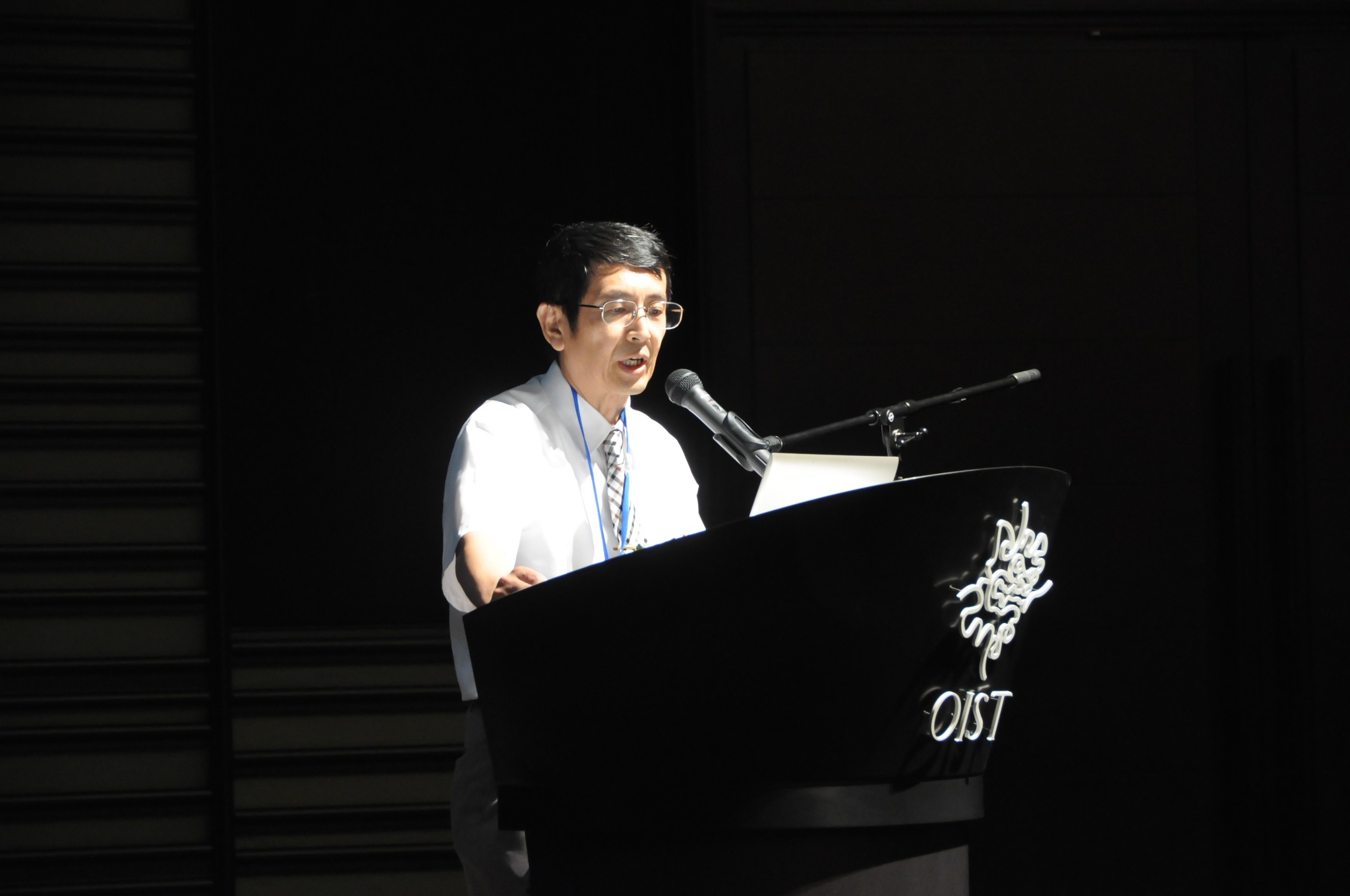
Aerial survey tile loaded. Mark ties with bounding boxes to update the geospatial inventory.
[602,431,647,555]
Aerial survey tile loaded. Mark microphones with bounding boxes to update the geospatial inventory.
[665,368,771,478]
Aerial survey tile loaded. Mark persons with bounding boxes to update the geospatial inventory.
[439,220,708,896]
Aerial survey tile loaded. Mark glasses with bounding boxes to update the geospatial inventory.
[554,300,684,330]
